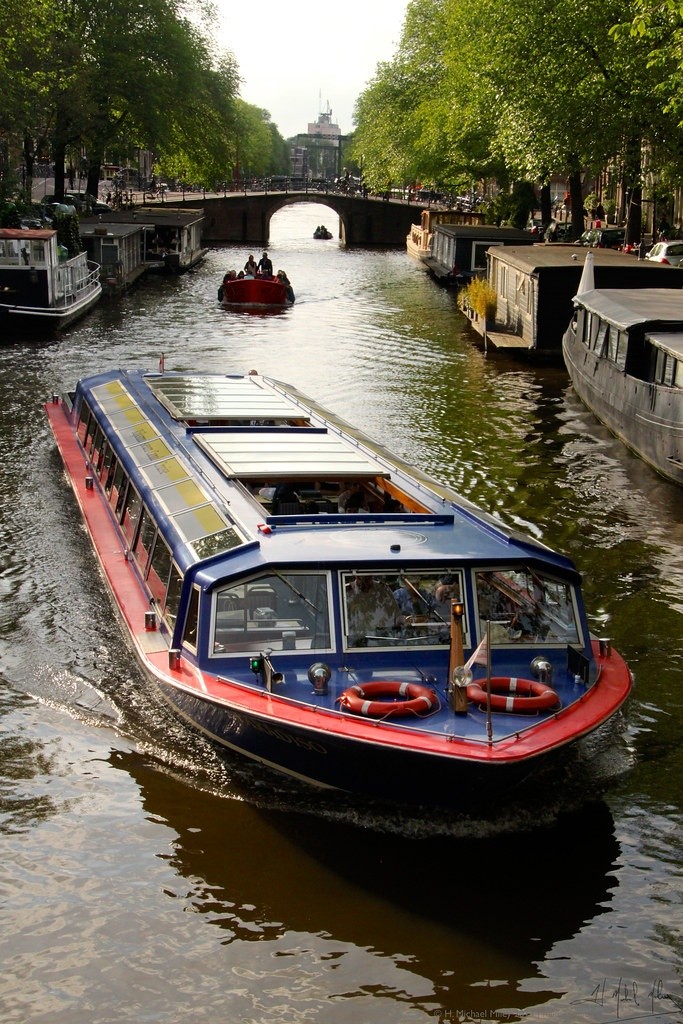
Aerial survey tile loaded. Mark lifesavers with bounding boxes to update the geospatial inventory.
[469,678,558,711]
[342,681,435,716]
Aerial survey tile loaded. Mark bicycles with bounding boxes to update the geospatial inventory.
[100,172,166,206]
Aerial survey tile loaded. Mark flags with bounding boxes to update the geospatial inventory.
[464,634,488,670]
[159,355,162,372]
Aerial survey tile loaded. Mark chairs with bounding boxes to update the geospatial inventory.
[274,498,303,514]
[215,585,278,623]
[308,498,331,513]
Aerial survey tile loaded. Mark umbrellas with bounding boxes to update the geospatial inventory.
[574,251,595,307]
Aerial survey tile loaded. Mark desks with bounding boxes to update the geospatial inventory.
[252,494,342,508]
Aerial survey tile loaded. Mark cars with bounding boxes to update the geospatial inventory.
[577,227,626,251]
[525,218,575,244]
[645,239,683,269]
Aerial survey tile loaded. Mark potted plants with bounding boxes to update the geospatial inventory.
[475,285,498,331]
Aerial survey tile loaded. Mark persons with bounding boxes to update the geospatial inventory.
[274,482,310,513]
[256,253,273,276]
[657,218,669,242]
[343,167,353,181]
[106,189,129,204]
[316,226,327,234]
[564,194,571,214]
[338,483,368,513]
[457,202,462,210]
[590,202,604,219]
[348,570,498,630]
[244,255,258,274]
[277,270,287,285]
[221,270,245,284]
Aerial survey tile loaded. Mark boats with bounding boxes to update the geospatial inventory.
[1,227,104,336]
[48,346,638,782]
[565,287,683,482]
[215,272,289,313]
